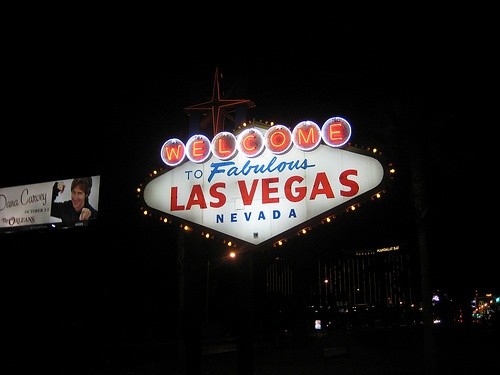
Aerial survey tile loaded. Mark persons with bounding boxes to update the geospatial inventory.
[51,176,98,222]
